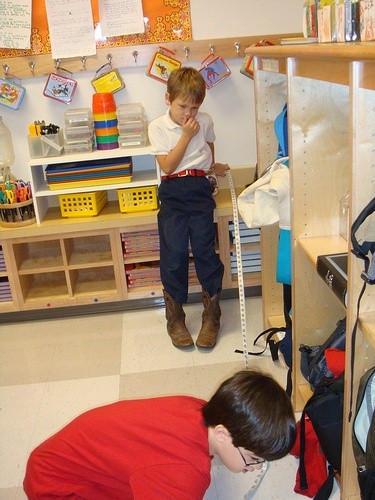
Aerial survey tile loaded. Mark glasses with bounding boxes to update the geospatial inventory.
[230,431,266,469]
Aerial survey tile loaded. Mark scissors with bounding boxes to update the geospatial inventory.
[0,180,35,223]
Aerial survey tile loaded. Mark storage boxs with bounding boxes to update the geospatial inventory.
[59,190,109,218]
[116,185,158,213]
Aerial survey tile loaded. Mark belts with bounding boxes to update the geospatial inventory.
[161,169,207,180]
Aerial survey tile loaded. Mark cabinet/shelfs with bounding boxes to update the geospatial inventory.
[0,166,262,314]
[28,143,161,223]
[243,39,375,500]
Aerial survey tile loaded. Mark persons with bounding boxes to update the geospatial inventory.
[23,367,297,500]
[148,67,231,348]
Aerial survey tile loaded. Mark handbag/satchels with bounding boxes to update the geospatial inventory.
[234,327,292,371]
[298,316,345,388]
[352,366,375,500]
[299,372,344,500]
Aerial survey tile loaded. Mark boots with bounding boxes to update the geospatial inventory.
[162,289,194,347]
[196,289,222,348]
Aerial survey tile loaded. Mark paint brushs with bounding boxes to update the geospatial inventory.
[40,124,59,134]
[28,120,45,136]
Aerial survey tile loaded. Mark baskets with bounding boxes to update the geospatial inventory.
[115,185,158,212]
[58,189,108,217]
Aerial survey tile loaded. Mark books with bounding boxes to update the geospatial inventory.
[230,249,261,273]
[316,252,349,309]
[281,0,375,44]
[228,220,260,245]
[0,282,13,302]
[121,228,217,288]
[0,251,6,272]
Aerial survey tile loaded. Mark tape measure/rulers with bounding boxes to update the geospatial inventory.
[227,168,269,497]
